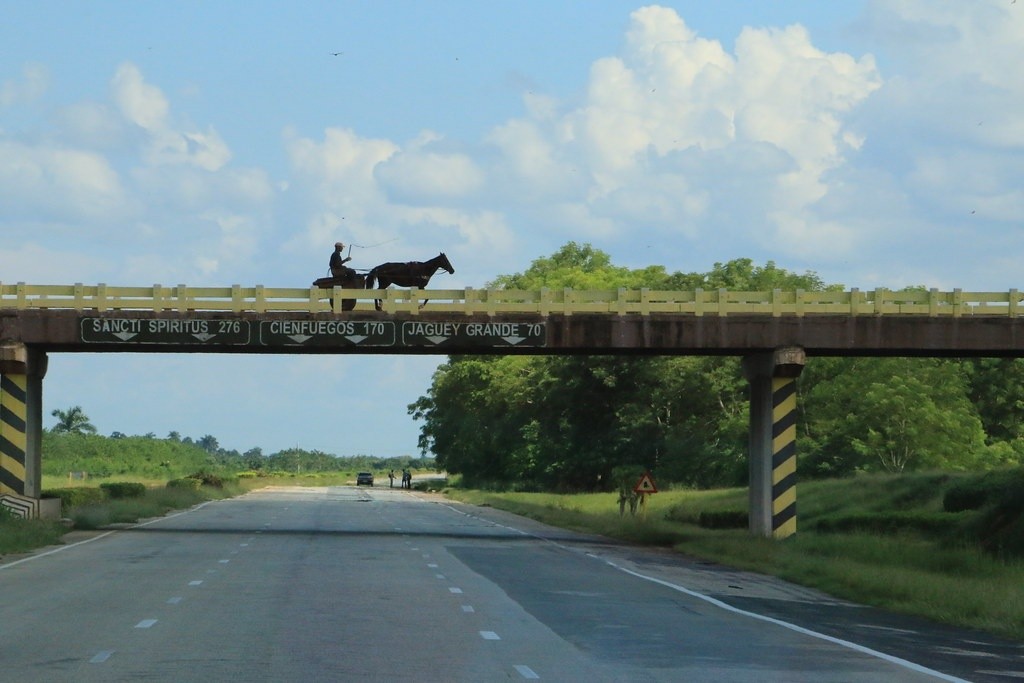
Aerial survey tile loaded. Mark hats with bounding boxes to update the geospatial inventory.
[335,242,346,248]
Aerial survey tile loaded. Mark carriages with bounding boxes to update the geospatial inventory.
[313,252,455,311]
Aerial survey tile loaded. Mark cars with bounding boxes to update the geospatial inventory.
[357,473,374,487]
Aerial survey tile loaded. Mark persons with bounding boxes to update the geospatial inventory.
[401,469,411,489]
[329,242,355,281]
[389,469,396,488]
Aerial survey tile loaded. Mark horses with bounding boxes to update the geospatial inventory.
[365,252,455,311]
